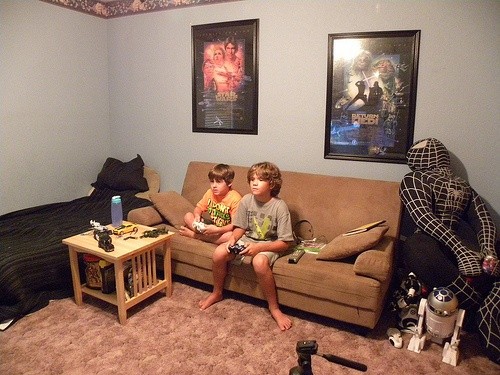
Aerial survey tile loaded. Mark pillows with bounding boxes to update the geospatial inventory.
[91,159,149,192]
[149,191,195,229]
[317,226,390,259]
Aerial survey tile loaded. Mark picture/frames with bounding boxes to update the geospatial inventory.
[323,29,422,165]
[191,19,260,135]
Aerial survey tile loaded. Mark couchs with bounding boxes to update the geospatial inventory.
[127,161,405,330]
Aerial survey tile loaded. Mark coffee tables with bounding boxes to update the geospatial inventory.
[63,221,176,324]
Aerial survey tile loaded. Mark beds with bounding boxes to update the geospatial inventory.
[0,167,161,332]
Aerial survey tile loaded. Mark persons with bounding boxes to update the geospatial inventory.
[202,35,243,93]
[199,162,296,330]
[400,136,500,365]
[179,164,243,245]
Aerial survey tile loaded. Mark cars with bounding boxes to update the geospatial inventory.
[113,224,134,235]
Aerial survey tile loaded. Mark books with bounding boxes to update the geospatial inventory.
[342,220,386,236]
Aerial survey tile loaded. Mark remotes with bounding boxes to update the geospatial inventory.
[287,249,305,263]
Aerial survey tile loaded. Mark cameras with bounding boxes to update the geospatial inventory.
[98,232,115,251]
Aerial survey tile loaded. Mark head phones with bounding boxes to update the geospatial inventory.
[292,219,316,246]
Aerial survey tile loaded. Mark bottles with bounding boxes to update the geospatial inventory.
[110,195,122,227]
